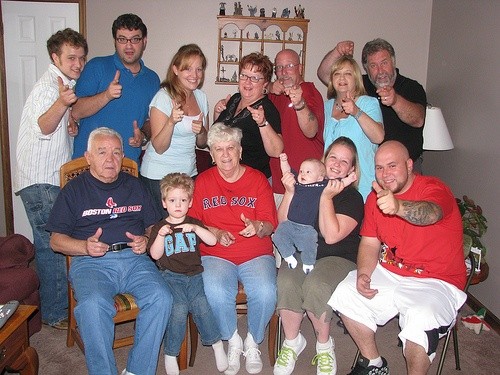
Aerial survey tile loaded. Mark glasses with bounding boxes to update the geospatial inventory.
[239,73,264,83]
[274,64,298,72]
[116,36,142,44]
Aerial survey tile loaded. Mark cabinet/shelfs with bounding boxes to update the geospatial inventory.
[215,15,310,85]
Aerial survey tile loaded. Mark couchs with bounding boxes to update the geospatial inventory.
[0,234,40,337]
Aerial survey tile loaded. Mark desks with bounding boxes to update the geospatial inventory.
[0,305,38,375]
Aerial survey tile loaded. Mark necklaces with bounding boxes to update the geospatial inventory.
[131,62,141,74]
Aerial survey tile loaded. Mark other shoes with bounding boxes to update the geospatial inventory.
[43,319,69,329]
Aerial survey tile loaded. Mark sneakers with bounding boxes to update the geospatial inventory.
[273,331,307,375]
[312,336,337,375]
[224,336,244,375]
[347,354,391,375]
[243,341,263,374]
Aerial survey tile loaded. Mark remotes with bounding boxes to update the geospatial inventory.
[0,300,19,327]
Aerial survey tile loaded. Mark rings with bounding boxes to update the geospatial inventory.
[226,244,228,245]
[218,107,220,109]
[293,95,295,98]
[247,232,249,235]
[385,97,387,101]
[139,248,141,251]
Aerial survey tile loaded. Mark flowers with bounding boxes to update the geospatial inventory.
[456,195,488,264]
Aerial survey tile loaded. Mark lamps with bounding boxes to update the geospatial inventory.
[422,106,455,150]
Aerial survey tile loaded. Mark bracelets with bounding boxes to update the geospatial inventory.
[355,110,362,119]
[294,100,305,110]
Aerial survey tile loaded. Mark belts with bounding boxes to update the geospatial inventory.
[106,243,132,253]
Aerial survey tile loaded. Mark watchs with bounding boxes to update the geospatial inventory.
[258,121,269,127]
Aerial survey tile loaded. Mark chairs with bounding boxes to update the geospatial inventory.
[351,251,475,375]
[189,283,279,367]
[61,158,188,370]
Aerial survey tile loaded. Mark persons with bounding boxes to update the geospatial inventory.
[272,152,358,274]
[45,127,173,375]
[317,37,427,170]
[70,13,161,170]
[273,136,365,375]
[328,141,468,375]
[140,44,209,198]
[267,49,324,209]
[15,28,89,330]
[321,55,385,204]
[186,122,279,375]
[212,51,284,190]
[147,173,229,375]
